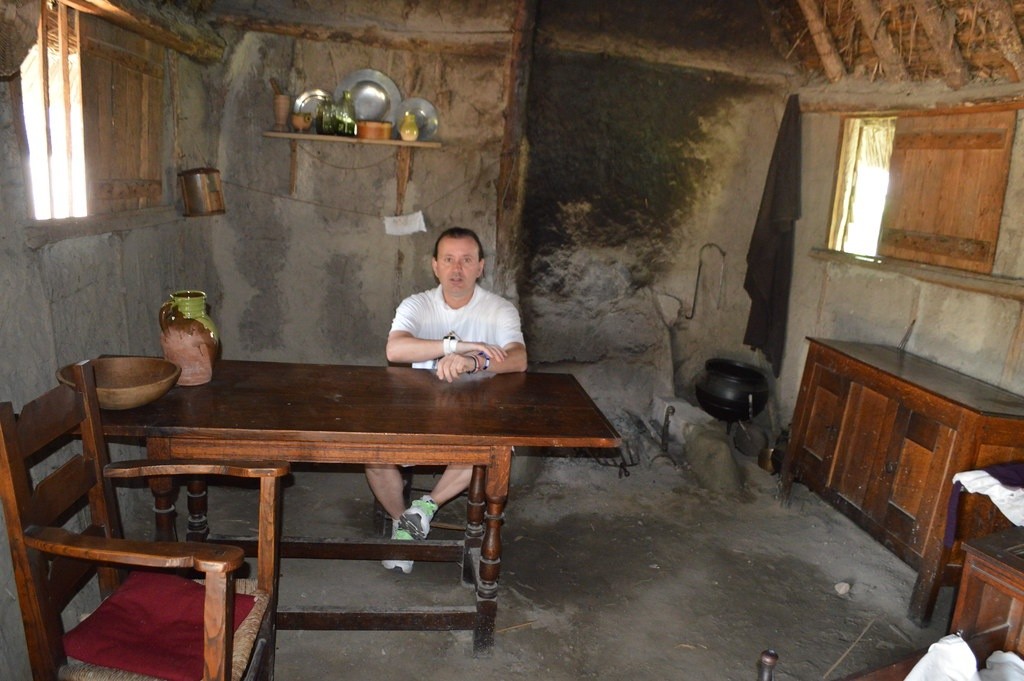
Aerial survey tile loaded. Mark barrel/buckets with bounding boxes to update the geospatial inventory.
[177,167,225,217]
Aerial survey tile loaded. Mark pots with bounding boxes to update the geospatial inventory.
[695,358,770,436]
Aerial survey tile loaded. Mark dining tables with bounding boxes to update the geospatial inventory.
[74,352,624,652]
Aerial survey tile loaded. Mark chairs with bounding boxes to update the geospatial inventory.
[0,362,279,681]
[375,331,483,541]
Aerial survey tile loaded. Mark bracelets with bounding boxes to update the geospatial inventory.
[443,333,490,375]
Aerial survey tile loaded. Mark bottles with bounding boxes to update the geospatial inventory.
[335,88,358,136]
[769,427,790,476]
[159,289,220,386]
[290,112,312,133]
[398,112,419,142]
[272,93,290,132]
[315,95,337,135]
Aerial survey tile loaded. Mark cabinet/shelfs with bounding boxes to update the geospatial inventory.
[770,335,1023,629]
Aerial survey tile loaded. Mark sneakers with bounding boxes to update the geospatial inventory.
[398,494,437,541]
[383,521,416,573]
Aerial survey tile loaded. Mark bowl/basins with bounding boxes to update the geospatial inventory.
[56,357,182,410]
[356,120,392,138]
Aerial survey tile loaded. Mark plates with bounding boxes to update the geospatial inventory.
[293,89,337,133]
[331,68,402,139]
[396,97,439,142]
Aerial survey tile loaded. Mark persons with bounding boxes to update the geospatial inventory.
[366,227,530,576]
